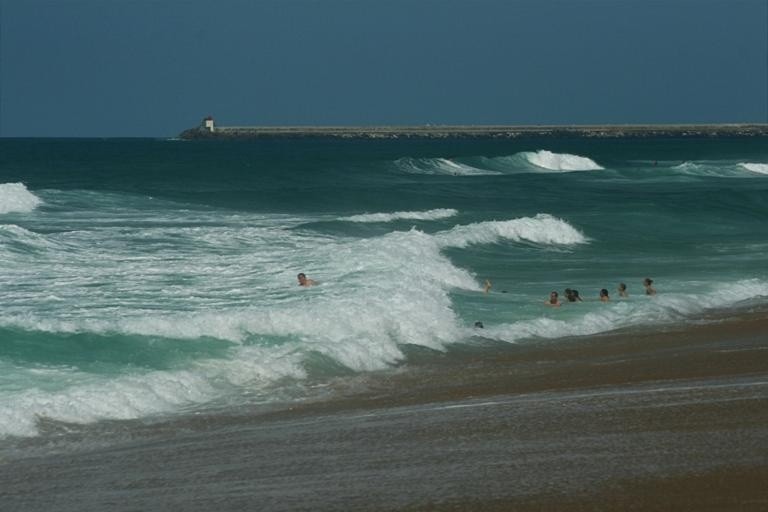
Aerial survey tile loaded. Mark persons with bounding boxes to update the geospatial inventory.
[546,277,584,308]
[473,321,484,328]
[618,283,629,298]
[295,273,317,288]
[600,288,610,303]
[485,279,492,293]
[642,278,655,295]
